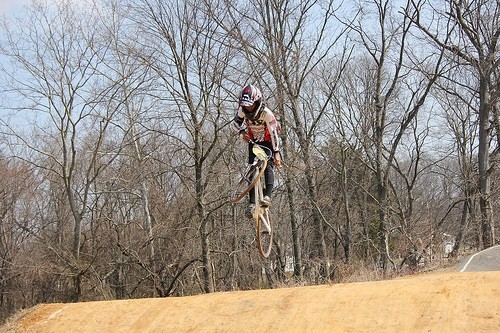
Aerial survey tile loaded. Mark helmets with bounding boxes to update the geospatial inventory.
[239,84,262,118]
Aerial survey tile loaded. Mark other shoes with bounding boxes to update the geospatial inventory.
[244,203,255,214]
[261,195,270,205]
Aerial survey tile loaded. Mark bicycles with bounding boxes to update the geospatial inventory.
[229,139,289,260]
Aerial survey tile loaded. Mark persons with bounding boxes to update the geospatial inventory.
[233,85,282,218]
